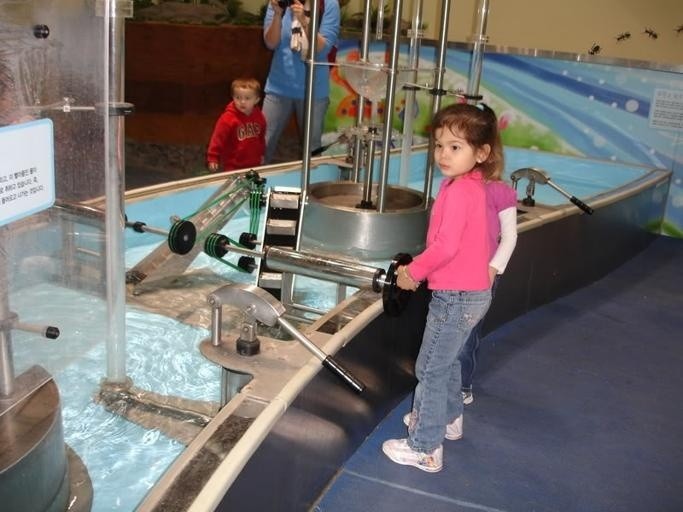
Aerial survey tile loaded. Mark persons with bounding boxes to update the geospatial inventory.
[206,76,267,171]
[459,131,517,404]
[262,0,340,165]
[382,103,497,472]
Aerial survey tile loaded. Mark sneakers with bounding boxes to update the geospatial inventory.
[382,439,443,472]
[461,391,473,404]
[403,412,463,440]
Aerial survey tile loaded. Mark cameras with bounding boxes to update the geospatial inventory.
[278,0,305,11]
